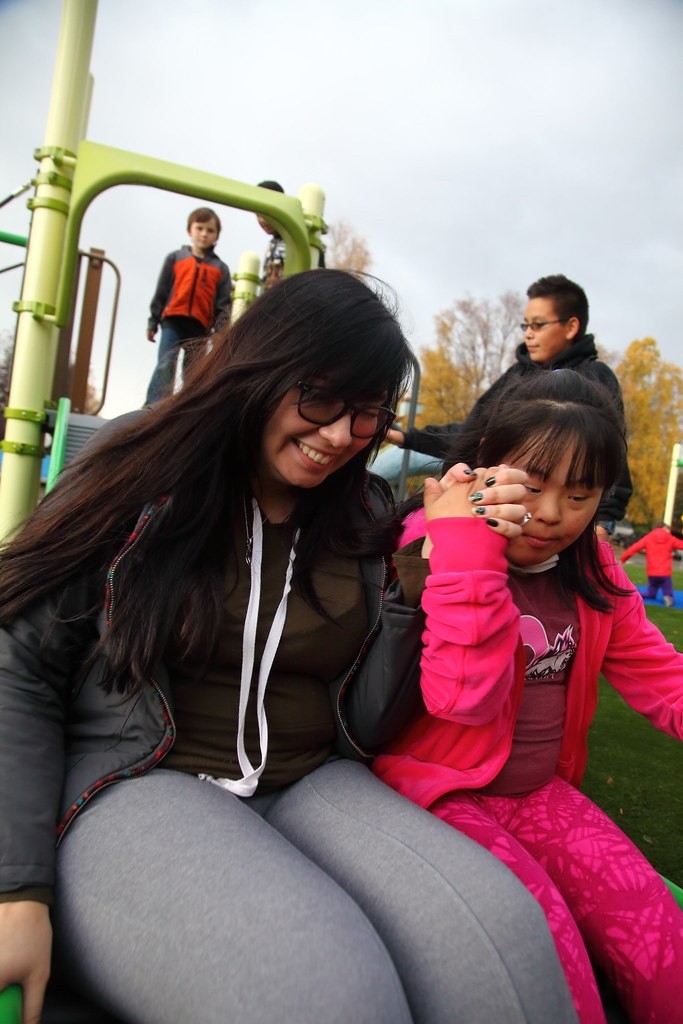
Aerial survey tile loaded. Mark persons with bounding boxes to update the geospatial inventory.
[144,208,232,409]
[384,275,633,541]
[363,369,682,1024]
[254,181,286,287]
[0,270,577,1024]
[620,521,683,606]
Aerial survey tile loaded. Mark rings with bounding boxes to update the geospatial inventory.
[520,512,531,525]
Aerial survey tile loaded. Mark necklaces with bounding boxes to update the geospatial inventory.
[243,495,272,566]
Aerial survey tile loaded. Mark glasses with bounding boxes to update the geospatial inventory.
[520,318,571,332]
[293,379,395,441]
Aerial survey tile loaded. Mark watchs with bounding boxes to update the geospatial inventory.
[597,520,614,535]
